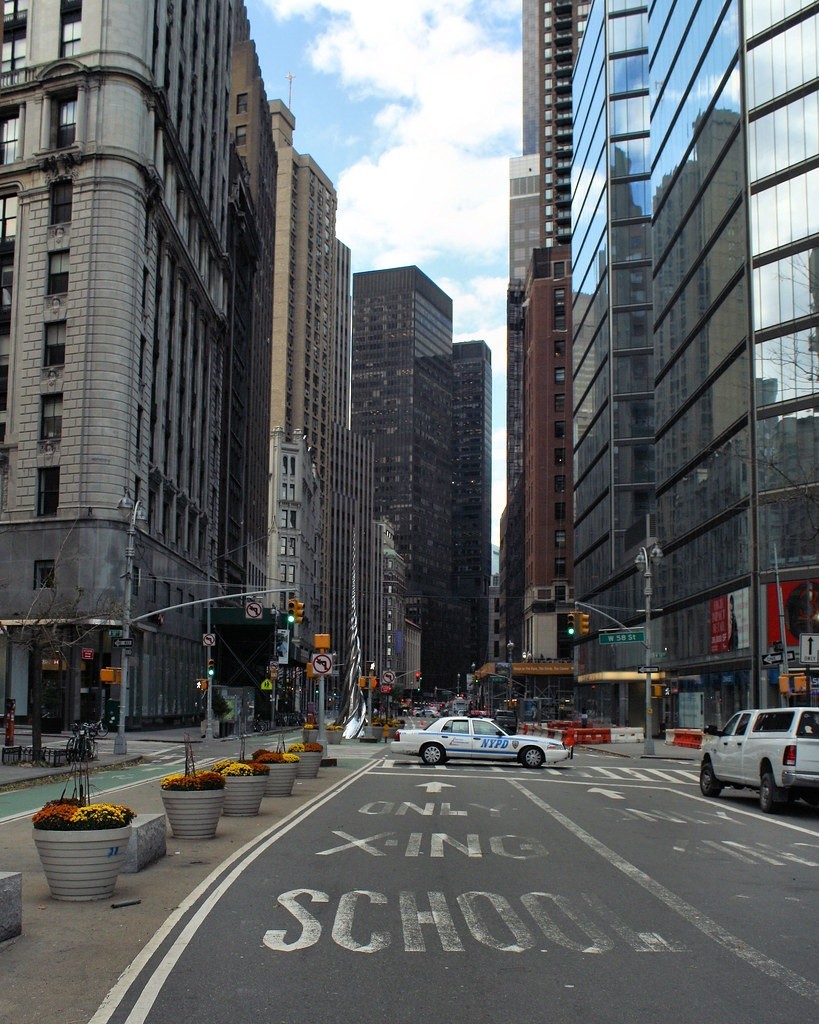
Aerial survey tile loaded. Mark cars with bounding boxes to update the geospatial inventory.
[413,702,467,716]
[390,716,573,769]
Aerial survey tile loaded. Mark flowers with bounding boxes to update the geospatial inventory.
[380,718,405,728]
[211,759,271,776]
[289,741,324,754]
[304,723,319,730]
[365,717,383,728]
[250,749,300,763]
[160,770,227,792]
[33,798,138,833]
[326,725,345,731]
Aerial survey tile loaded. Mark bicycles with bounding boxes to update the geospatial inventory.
[66,712,108,762]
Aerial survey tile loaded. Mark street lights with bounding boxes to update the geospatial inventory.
[471,663,475,701]
[635,540,664,755]
[456,674,460,693]
[506,640,515,708]
[269,602,279,729]
[114,486,147,754]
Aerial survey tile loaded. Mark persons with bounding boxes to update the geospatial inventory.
[531,703,537,721]
[379,706,385,718]
[728,595,739,652]
[582,704,588,728]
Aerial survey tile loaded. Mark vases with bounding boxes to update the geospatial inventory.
[261,763,298,797]
[326,730,344,745]
[289,752,322,778]
[160,787,227,838]
[387,728,399,738]
[33,826,132,902]
[221,775,268,817]
[302,730,319,742]
[398,724,405,729]
[364,727,384,741]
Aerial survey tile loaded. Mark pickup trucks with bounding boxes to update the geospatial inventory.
[494,710,518,732]
[700,707,819,814]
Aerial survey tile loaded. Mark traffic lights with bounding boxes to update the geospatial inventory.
[457,693,465,697]
[778,676,789,694]
[567,614,575,636]
[416,673,420,681]
[420,672,423,681]
[208,659,215,678]
[287,600,295,624]
[653,685,662,697]
[578,613,590,634]
[358,677,365,687]
[365,661,375,670]
[194,680,207,690]
[296,600,305,623]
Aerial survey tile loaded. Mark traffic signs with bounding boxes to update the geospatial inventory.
[762,650,795,664]
[112,637,133,647]
[637,666,660,673]
[663,687,673,697]
